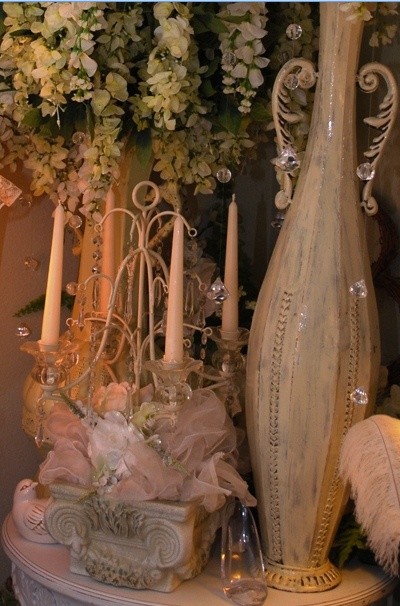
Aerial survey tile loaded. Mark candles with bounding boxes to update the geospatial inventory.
[99,182,117,313]
[220,196,242,334]
[161,210,189,368]
[39,200,65,345]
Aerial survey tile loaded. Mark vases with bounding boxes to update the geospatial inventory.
[244,2,398,594]
[22,141,161,494]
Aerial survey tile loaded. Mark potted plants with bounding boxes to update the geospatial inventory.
[0,1,400,349]
